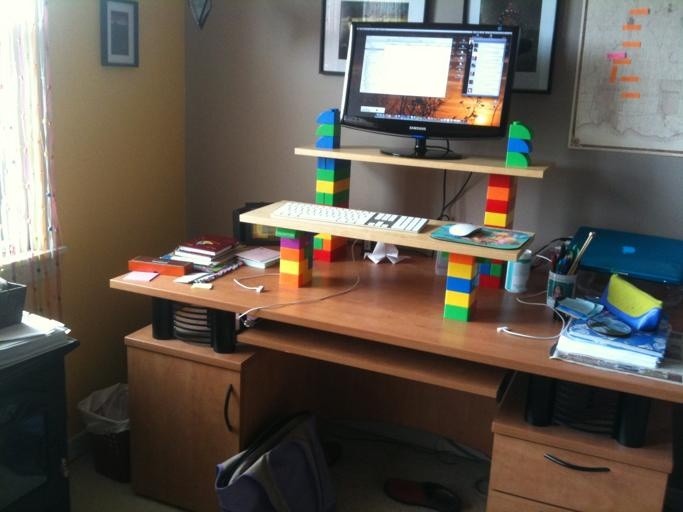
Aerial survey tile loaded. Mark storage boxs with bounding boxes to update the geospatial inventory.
[571,225,682,306]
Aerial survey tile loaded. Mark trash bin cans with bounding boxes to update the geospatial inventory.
[78,384,133,482]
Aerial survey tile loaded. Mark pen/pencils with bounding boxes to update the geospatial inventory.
[192,261,242,282]
[548,242,580,275]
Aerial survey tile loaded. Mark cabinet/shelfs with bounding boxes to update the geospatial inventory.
[483,434,671,511]
[126,345,308,511]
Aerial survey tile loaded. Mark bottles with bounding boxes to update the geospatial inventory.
[504,248,533,293]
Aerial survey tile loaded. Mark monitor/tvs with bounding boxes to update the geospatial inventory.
[339,21,522,160]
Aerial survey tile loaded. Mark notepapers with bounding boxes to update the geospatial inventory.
[234,246,279,269]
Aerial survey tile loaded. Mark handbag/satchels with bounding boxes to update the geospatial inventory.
[215,410,338,511]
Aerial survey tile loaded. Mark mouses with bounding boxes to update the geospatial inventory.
[449,222,481,237]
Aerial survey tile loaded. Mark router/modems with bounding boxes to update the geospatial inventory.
[360,241,376,257]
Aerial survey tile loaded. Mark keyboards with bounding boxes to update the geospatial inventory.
[272,200,430,234]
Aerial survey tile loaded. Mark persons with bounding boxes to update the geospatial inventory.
[551,284,564,300]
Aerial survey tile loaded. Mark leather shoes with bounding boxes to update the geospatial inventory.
[382,478,461,511]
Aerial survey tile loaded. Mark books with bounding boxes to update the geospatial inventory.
[557,316,663,369]
[159,234,282,273]
[566,311,672,357]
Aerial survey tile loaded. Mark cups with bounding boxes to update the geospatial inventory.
[546,268,580,308]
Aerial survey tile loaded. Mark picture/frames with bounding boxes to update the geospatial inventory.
[318,1,559,96]
[98,1,140,67]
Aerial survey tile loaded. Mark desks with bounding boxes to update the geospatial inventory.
[109,238,683,511]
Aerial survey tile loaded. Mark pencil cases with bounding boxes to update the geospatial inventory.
[128,256,193,276]
